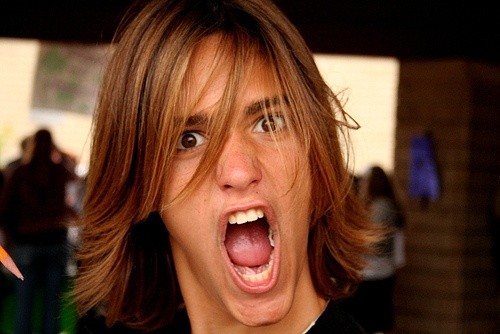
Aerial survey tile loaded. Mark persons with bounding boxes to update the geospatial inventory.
[346,166,406,334]
[0,129,78,333]
[71,1,399,334]
